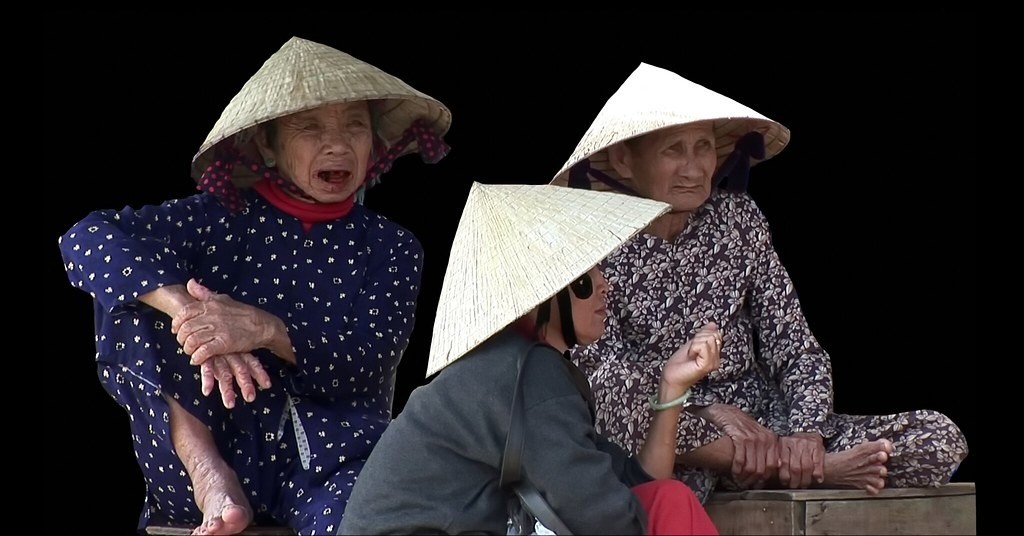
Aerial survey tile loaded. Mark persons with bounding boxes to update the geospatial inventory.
[59,37,452,536]
[337,181,722,535]
[548,62,968,507]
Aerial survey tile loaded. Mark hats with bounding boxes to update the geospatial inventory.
[552,60,790,193]
[425,181,673,383]
[192,37,452,189]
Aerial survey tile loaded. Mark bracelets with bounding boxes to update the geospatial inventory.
[649,390,690,410]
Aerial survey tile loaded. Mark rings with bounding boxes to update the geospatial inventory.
[716,339,721,345]
